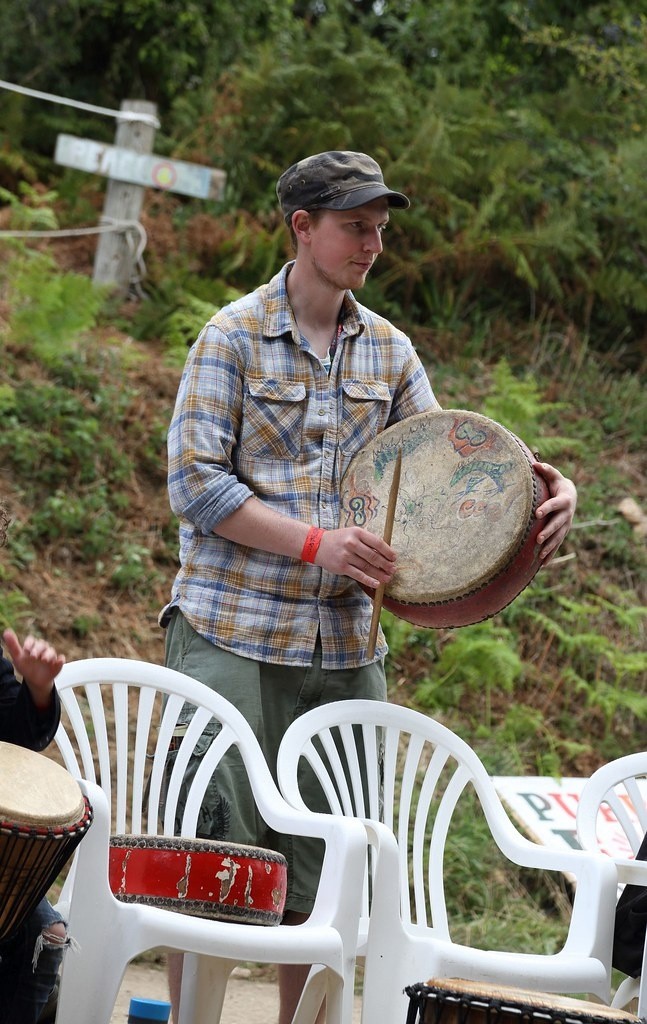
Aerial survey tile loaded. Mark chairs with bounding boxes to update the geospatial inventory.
[49,657,647,1024]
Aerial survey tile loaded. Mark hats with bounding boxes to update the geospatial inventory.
[276,150,410,220]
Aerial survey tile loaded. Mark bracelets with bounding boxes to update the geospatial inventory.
[297,524,325,564]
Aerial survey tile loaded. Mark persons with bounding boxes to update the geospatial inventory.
[158,149,576,1024]
[0,627,71,1024]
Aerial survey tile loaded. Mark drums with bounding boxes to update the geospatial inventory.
[340,406,557,630]
[405,977,645,1024]
[0,739,93,958]
[109,834,287,928]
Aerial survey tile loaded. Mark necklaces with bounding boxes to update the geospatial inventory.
[286,286,341,379]
